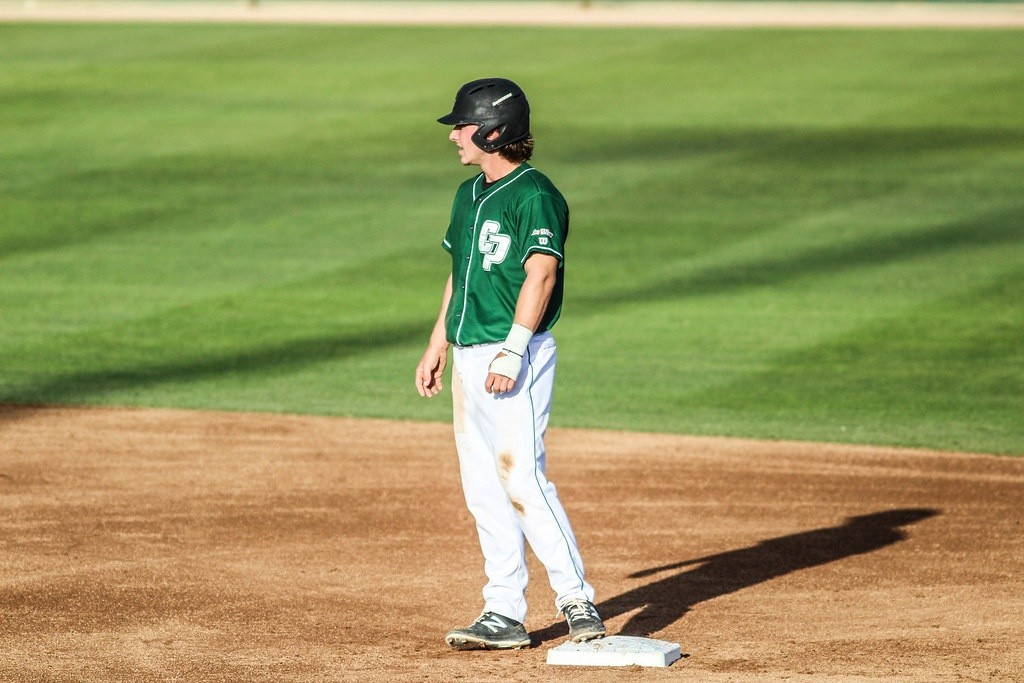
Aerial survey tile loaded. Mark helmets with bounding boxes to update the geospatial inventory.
[436,78,530,153]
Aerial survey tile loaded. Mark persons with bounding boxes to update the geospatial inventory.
[414,78,608,651]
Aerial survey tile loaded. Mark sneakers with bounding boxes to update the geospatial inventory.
[556,598,606,643]
[445,612,531,650]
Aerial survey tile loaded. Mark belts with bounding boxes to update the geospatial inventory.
[455,343,472,347]
